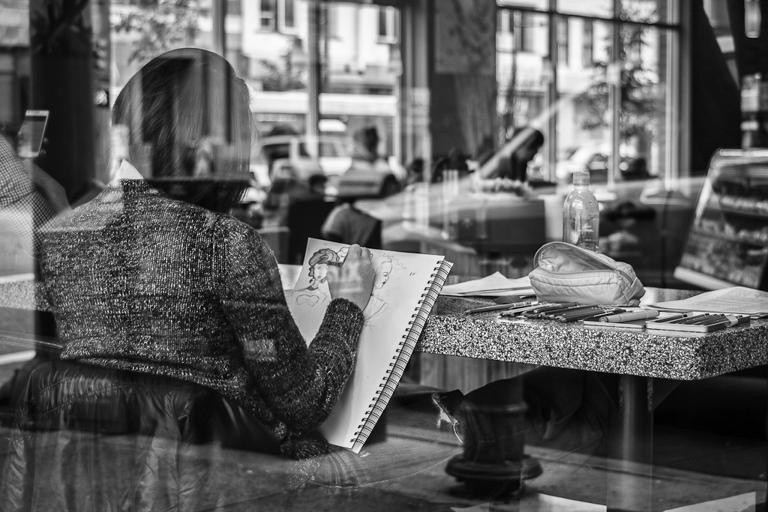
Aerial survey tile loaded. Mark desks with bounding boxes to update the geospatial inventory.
[414,276,768,512]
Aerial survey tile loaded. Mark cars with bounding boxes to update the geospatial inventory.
[357,67,684,277]
[250,133,407,204]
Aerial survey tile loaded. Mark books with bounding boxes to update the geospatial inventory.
[287,236,454,456]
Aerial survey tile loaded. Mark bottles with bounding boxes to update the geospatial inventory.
[563,172,601,253]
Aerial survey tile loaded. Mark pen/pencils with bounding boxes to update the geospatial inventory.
[327,261,342,267]
[457,286,531,294]
[463,299,743,326]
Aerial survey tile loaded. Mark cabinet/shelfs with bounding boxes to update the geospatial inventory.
[673,147,768,290]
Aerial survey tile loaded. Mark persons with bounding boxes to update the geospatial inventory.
[0,135,72,259]
[31,43,378,458]
[317,124,408,202]
[472,124,545,180]
[362,255,393,321]
[306,248,341,291]
